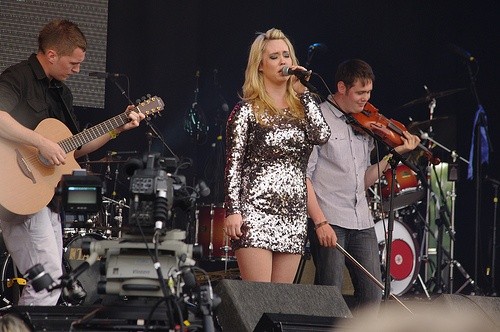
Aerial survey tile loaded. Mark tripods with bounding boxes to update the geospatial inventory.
[419,185,485,299]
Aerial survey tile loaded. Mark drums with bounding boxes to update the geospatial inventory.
[371,217,421,297]
[0,207,111,313]
[375,161,427,212]
[197,202,239,264]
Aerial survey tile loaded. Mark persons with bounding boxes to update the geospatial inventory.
[225,26,332,284]
[308,60,421,307]
[0,18,146,306]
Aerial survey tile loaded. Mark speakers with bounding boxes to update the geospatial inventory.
[211,278,355,332]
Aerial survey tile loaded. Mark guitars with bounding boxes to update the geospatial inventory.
[0,93,165,217]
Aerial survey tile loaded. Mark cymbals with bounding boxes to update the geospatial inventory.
[79,155,129,164]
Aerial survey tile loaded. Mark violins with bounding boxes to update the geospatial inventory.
[348,101,440,165]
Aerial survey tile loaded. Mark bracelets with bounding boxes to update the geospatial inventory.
[383,156,390,161]
[109,130,117,138]
[315,221,328,230]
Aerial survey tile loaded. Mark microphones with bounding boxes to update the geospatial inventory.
[90,72,122,78]
[282,66,317,76]
[198,181,210,197]
[462,49,477,64]
